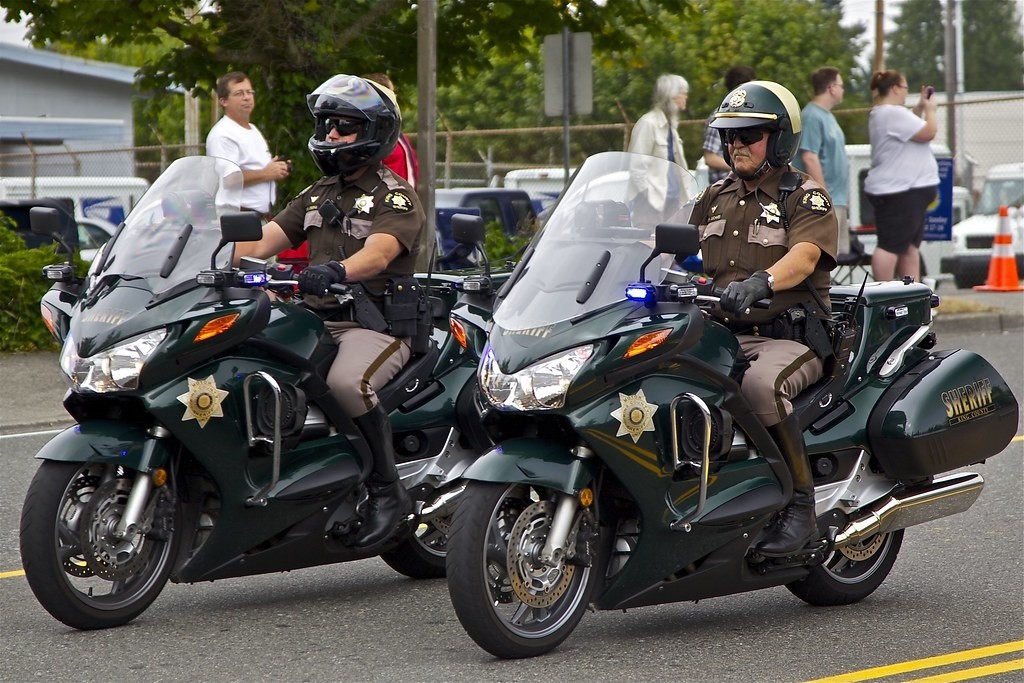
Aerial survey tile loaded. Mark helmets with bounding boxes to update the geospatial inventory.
[709,79,802,169]
[306,74,401,176]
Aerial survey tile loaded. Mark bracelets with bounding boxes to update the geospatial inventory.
[765,270,775,290]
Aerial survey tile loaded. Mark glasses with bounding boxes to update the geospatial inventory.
[232,88,255,98]
[726,129,770,145]
[834,82,843,88]
[323,118,363,136]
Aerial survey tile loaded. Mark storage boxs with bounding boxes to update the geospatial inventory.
[824,278,940,347]
[867,350,1018,480]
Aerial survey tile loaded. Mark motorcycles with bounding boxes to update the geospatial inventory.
[446,153,1018,658]
[17,153,506,631]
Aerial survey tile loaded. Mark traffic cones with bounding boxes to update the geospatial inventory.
[973,206,1024,291]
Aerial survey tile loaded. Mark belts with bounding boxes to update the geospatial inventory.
[317,305,356,321]
[725,323,794,339]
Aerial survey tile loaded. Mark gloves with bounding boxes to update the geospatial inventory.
[298,260,346,299]
[720,269,776,316]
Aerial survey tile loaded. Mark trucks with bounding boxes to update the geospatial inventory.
[0,176,149,260]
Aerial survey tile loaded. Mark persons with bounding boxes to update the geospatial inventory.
[613,81,838,557]
[791,66,850,255]
[863,71,941,317]
[231,74,426,552]
[206,71,292,265]
[624,74,689,236]
[363,73,419,192]
[703,65,756,185]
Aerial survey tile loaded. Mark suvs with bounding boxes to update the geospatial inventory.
[845,142,1024,289]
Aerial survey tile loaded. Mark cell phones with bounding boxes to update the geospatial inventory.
[927,86,934,99]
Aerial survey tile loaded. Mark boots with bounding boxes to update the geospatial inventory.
[352,401,415,554]
[755,410,821,557]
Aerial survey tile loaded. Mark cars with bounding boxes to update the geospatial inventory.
[434,167,577,267]
